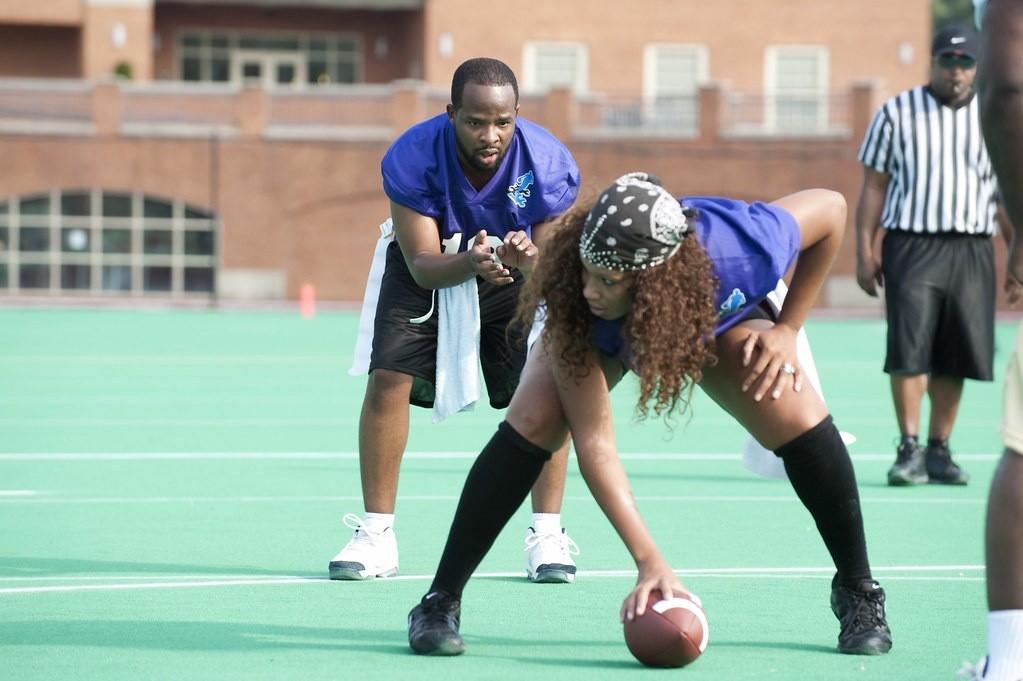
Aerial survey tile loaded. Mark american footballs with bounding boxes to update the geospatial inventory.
[624,588,710,669]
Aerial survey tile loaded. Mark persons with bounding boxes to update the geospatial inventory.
[395,167,897,656]
[327,58,584,588]
[961,1,1023,681]
[855,25,1023,488]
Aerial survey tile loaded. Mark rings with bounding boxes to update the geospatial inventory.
[778,363,795,375]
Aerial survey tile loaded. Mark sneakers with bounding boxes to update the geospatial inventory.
[328,514,399,579]
[831,572,893,655]
[409,589,467,657]
[890,439,968,485]
[524,525,581,583]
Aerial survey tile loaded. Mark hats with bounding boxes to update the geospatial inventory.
[579,170,695,272]
[931,23,979,60]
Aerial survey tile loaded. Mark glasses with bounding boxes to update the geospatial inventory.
[933,52,978,70]
